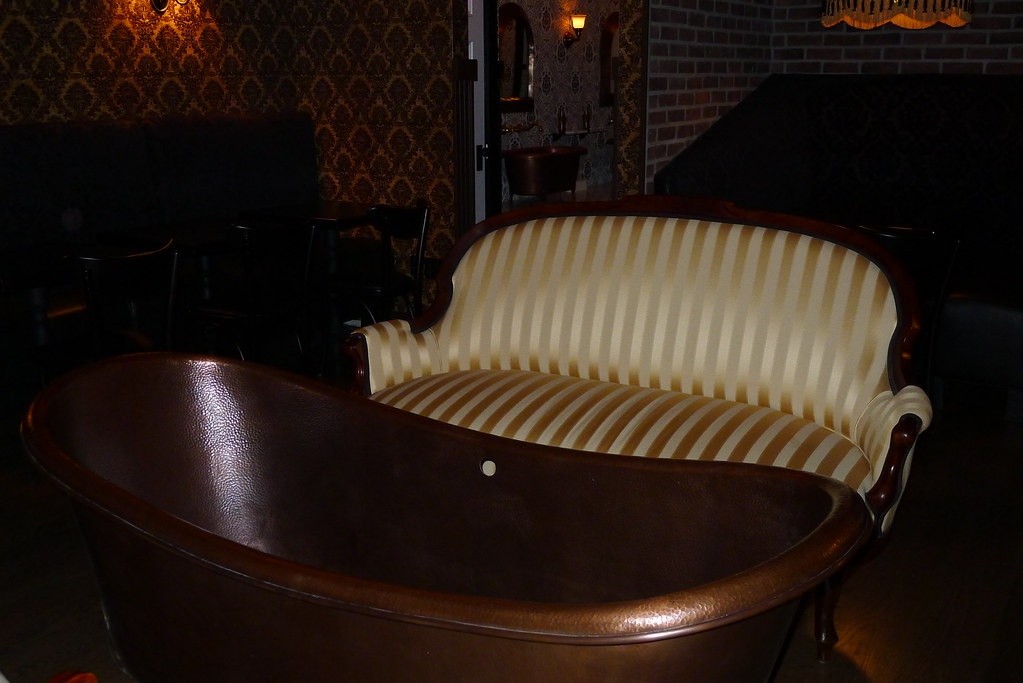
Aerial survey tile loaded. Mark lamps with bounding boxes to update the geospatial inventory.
[563,14,587,49]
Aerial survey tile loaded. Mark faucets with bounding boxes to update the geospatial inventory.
[519,112,544,133]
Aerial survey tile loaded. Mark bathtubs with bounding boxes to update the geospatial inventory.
[13,351,876,683]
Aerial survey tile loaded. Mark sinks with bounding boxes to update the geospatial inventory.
[503,145,588,197]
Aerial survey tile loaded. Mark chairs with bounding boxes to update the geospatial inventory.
[33,203,432,394]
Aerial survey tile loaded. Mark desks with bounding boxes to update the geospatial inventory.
[19,350,872,683]
[0,204,341,426]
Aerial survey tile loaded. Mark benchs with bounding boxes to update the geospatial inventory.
[342,197,933,669]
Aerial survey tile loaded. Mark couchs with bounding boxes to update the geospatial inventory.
[923,237,1023,424]
